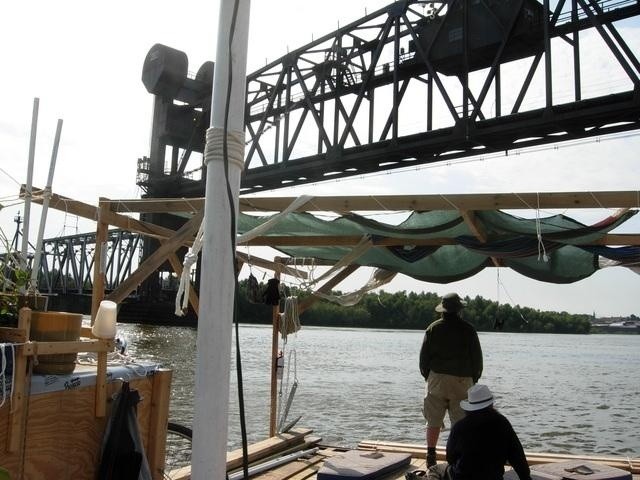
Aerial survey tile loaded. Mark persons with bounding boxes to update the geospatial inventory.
[406,383,532,480]
[418,293,484,470]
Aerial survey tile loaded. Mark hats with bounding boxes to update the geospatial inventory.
[460,385,502,412]
[434,292,468,313]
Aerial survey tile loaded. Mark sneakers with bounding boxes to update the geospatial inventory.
[426,452,436,468]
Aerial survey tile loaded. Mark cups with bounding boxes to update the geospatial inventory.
[91,300,118,340]
